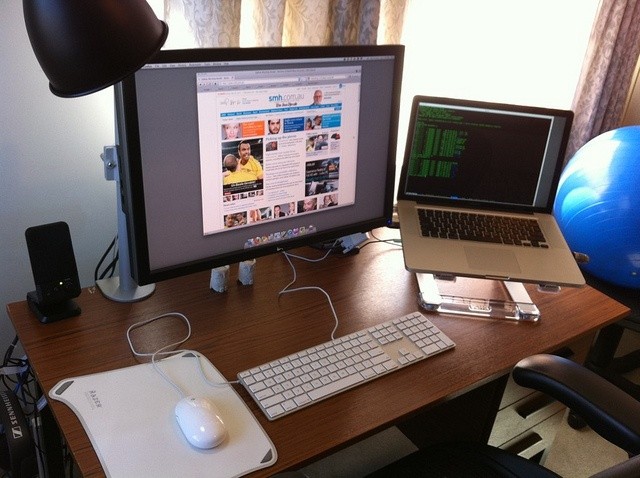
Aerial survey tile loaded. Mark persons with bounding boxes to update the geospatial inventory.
[328,193,337,206]
[310,90,324,105]
[237,213,246,226]
[223,154,257,186]
[306,136,316,152]
[237,142,262,179]
[273,205,285,219]
[223,123,240,140]
[305,119,312,129]
[314,115,322,127]
[225,215,236,228]
[315,135,330,151]
[303,198,316,211]
[319,196,331,208]
[268,119,280,135]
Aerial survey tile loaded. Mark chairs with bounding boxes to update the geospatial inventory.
[355,352,639,478]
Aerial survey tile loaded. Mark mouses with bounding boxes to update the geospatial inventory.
[174,394,229,450]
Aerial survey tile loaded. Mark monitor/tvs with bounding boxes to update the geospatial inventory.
[95,44,405,303]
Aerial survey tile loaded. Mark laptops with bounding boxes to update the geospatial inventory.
[396,95,586,288]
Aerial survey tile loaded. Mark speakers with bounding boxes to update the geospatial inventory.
[24,220,82,324]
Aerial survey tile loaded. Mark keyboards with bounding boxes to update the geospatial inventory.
[237,310,457,421]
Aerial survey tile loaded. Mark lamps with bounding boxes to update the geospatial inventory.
[22,0,170,99]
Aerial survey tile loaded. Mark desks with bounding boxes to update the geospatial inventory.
[6,218,631,478]
